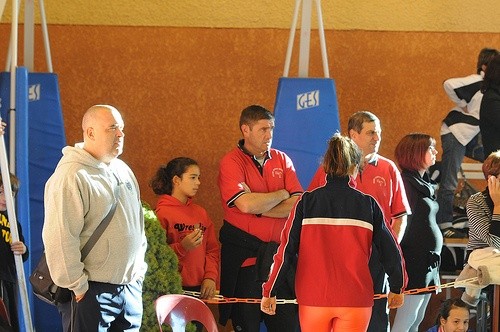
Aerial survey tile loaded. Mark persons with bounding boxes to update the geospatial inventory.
[479,54,500,159]
[425,299,470,332]
[391,133,443,332]
[218,105,304,332]
[463,149,500,332]
[260,132,409,332]
[152,157,220,332]
[320,111,413,332]
[0,118,29,332]
[436,48,500,239]
[42,104,148,332]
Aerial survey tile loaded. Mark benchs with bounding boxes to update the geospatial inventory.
[441,163,485,299]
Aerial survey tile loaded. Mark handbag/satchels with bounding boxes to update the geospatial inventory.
[30,249,78,307]
[454,165,478,211]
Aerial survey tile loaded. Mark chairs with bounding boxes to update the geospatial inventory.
[154,294,219,332]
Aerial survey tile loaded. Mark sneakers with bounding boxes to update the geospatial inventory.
[441,227,468,239]
